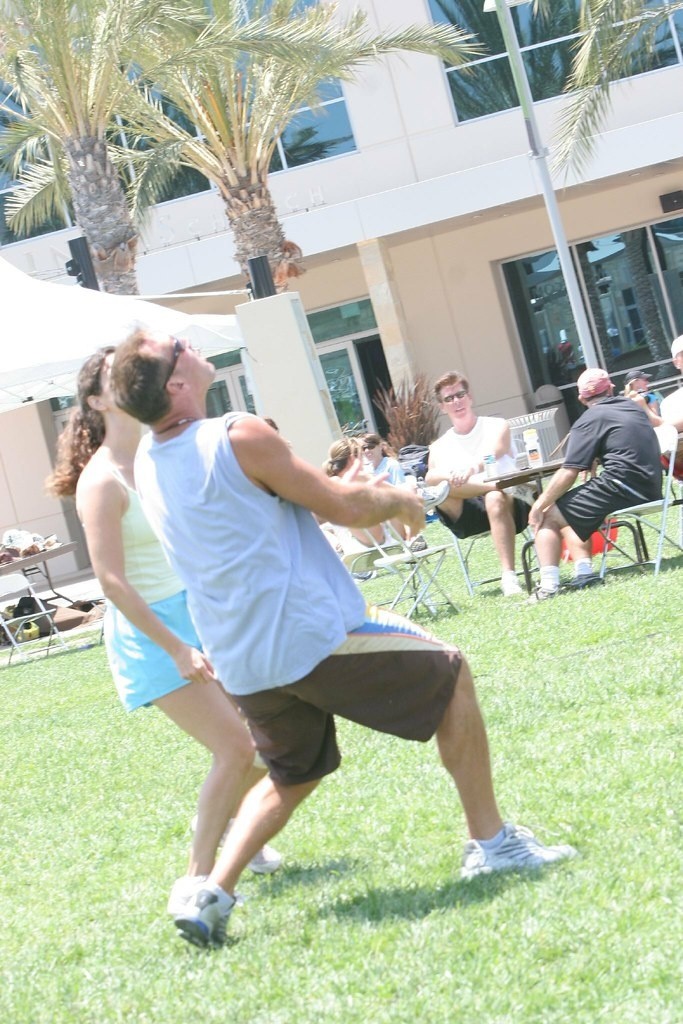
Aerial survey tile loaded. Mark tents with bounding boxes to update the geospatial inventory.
[0,256,263,422]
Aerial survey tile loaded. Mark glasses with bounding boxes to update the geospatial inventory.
[442,390,468,403]
[164,339,185,390]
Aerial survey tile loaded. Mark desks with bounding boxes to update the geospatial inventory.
[473,459,643,595]
[0,541,93,651]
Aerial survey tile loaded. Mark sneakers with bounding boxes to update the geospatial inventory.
[190,810,282,874]
[174,885,237,950]
[461,825,578,878]
[168,877,209,917]
[415,480,451,513]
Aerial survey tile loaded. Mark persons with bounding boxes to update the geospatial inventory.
[319,433,428,564]
[106,327,577,951]
[425,371,540,596]
[525,367,663,604]
[44,345,281,919]
[660,335,683,471]
[624,370,662,425]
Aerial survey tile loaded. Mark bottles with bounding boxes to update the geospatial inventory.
[482,440,498,478]
[523,429,544,468]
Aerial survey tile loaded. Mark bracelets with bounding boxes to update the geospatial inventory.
[473,464,479,475]
[158,417,195,434]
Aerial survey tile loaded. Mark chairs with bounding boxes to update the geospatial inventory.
[0,575,72,666]
[325,424,683,620]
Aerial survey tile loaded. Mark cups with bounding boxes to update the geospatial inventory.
[515,452,529,468]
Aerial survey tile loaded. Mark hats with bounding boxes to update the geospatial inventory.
[577,368,616,399]
[671,335,683,358]
[362,441,377,451]
[625,370,652,382]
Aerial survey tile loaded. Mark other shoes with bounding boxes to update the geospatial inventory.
[499,575,523,596]
[525,585,559,602]
[562,575,596,589]
[405,540,428,564]
